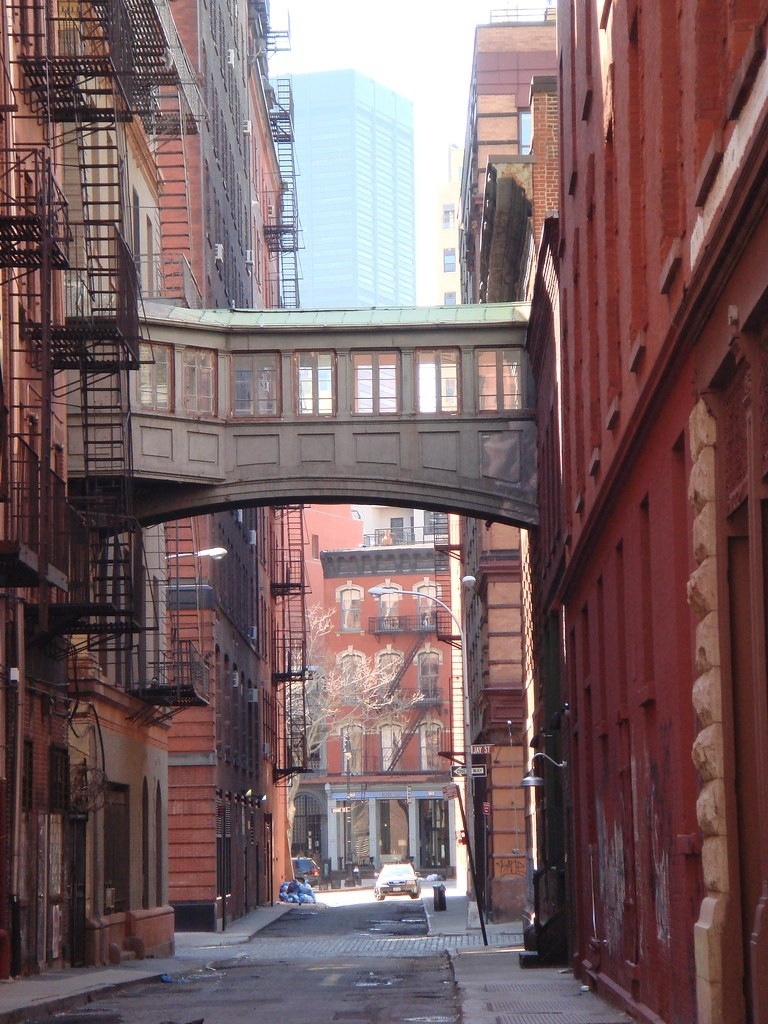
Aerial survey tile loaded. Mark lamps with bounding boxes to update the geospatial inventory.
[518,752,568,787]
[242,788,252,798]
[256,794,267,801]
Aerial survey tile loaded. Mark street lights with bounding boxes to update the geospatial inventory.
[367,586,483,931]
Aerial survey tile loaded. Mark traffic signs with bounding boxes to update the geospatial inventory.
[450,763,488,778]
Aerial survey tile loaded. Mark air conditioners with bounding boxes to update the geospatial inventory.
[267,204,275,218]
[248,687,259,703]
[249,529,257,545]
[250,625,258,639]
[243,120,252,138]
[245,249,254,265]
[264,741,272,756]
[233,672,240,688]
[237,509,243,522]
[227,49,235,69]
[214,241,224,262]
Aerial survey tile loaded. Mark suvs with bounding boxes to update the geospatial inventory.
[290,857,319,887]
[374,864,421,901]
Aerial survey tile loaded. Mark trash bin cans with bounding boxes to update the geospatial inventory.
[433,884,447,911]
